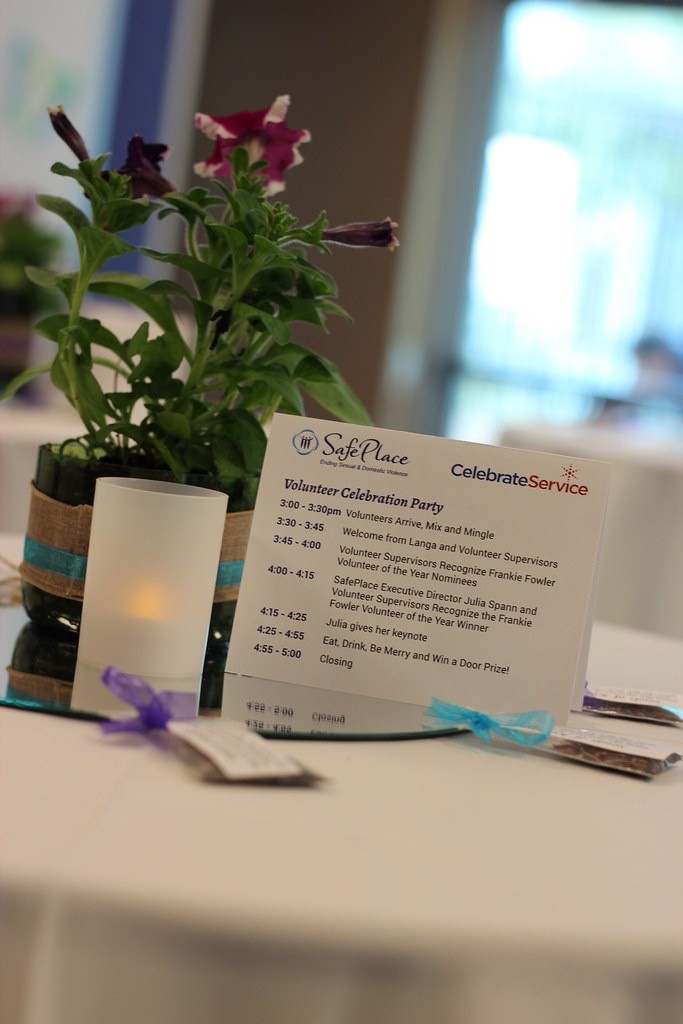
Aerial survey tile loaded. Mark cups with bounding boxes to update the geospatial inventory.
[70,476,229,719]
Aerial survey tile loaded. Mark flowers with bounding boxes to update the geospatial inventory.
[0,188,64,314]
[24,91,403,510]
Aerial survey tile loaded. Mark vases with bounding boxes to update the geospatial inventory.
[0,311,34,397]
[5,622,232,722]
[21,443,255,681]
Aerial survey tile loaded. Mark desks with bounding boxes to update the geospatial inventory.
[498,420,683,640]
[0,533,683,1024]
